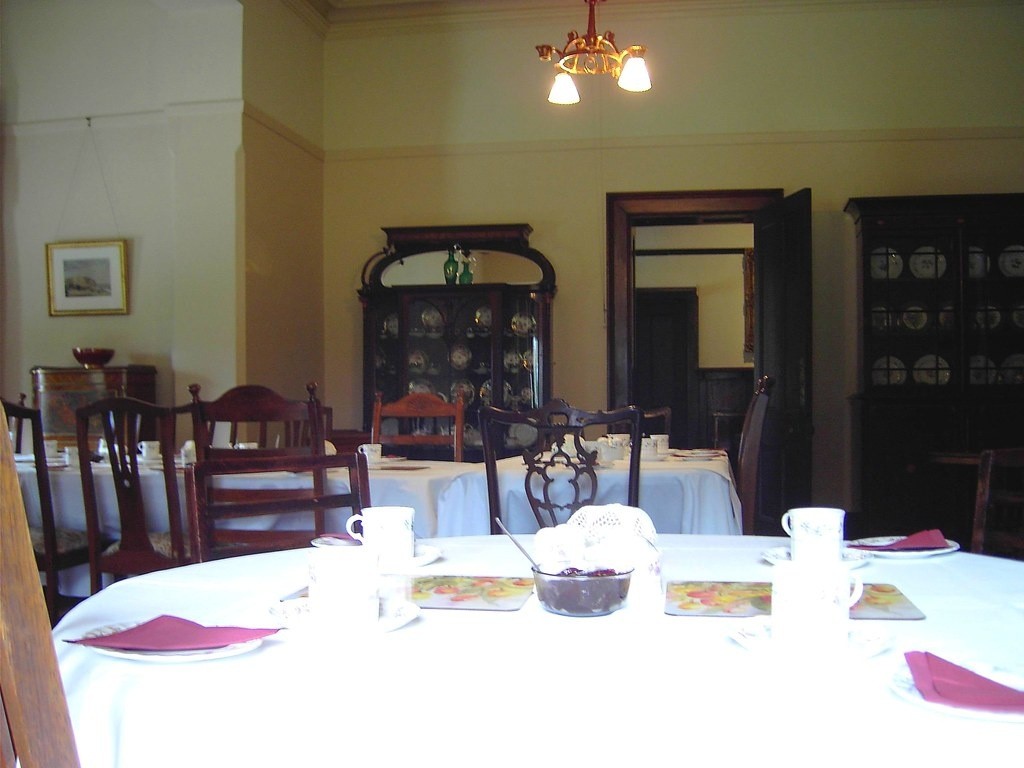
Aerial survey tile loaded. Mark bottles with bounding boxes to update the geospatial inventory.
[444,243,473,285]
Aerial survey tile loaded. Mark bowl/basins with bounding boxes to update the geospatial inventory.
[529,559,639,617]
[72,347,114,368]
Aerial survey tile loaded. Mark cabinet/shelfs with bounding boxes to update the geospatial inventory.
[843,193,1024,538]
[41,365,157,443]
[358,223,560,465]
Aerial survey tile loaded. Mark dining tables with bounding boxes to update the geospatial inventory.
[52,534,1024,768]
[437,430,744,540]
[13,449,472,599]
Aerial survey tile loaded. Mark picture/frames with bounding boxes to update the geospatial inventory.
[43,237,129,317]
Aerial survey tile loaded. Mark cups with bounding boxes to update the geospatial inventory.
[780,506,846,561]
[344,507,417,562]
[39,437,431,471]
[785,560,862,647]
[551,432,676,462]
[311,544,380,632]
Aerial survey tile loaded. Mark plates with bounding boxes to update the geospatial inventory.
[867,243,1024,387]
[378,307,534,410]
[677,447,721,462]
[81,532,1024,734]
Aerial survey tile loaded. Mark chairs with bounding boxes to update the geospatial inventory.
[0,377,768,621]
[969,445,1024,563]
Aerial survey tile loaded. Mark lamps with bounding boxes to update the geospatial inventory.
[535,0,651,105]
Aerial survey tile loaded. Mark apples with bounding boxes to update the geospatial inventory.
[411,577,532,602]
[672,583,903,613]
